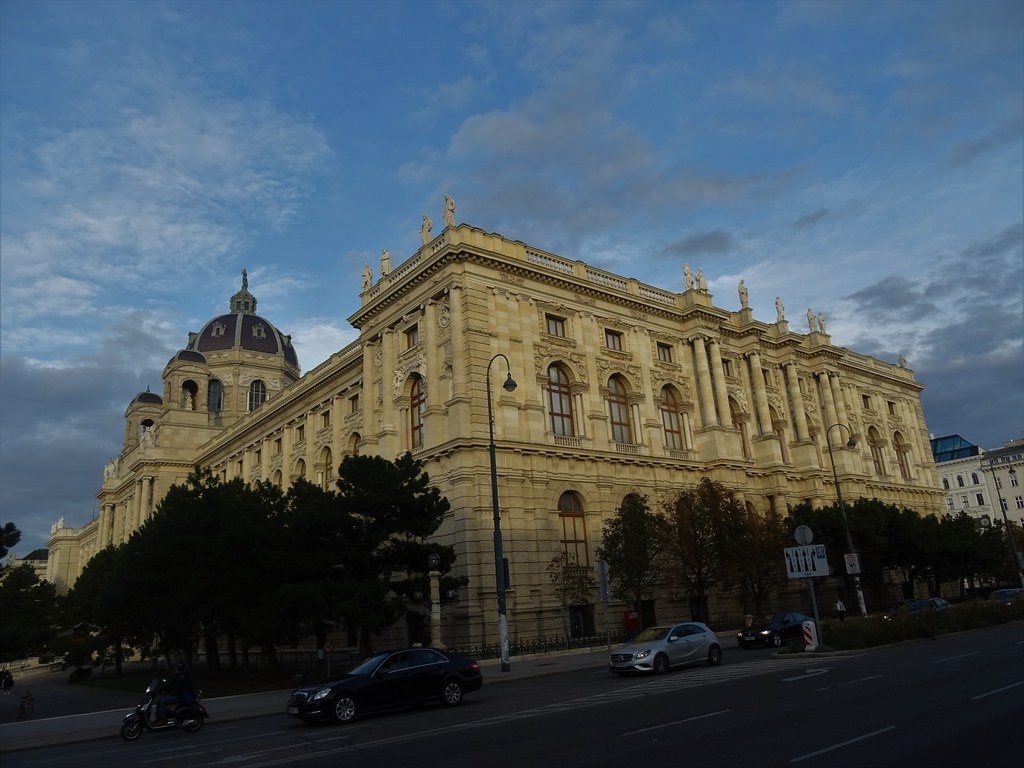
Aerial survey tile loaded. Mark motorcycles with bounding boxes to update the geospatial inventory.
[121,673,211,741]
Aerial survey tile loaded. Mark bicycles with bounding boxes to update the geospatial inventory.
[17,691,37,719]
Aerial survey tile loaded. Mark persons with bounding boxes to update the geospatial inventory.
[738,279,749,309]
[818,312,826,333]
[695,267,708,294]
[965,585,975,597]
[103,462,117,480]
[361,263,372,292]
[418,213,433,244]
[807,308,817,333]
[831,597,848,623]
[381,249,390,278]
[897,355,908,368]
[50,516,65,536]
[684,264,694,291]
[140,429,153,449]
[151,661,196,727]
[775,296,785,322]
[0,669,14,693]
[442,194,456,228]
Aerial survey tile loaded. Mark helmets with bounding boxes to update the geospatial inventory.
[177,661,185,671]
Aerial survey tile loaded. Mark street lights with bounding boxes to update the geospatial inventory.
[485,353,519,670]
[825,424,870,619]
[988,456,1023,587]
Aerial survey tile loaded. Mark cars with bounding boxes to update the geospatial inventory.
[880,598,951,630]
[608,621,724,676]
[983,589,1024,614]
[288,644,485,728]
[736,611,815,650]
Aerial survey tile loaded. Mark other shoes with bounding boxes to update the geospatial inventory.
[4,692,6,695]
[8,692,9,694]
[152,718,165,726]
